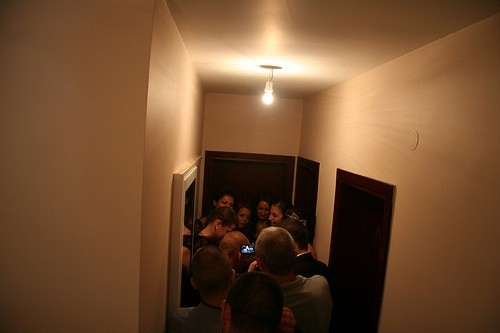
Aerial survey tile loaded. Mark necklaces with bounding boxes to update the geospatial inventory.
[200,231,207,236]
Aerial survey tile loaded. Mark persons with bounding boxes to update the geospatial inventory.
[166,191,333,333]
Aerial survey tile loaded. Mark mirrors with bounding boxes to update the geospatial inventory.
[167,154,204,319]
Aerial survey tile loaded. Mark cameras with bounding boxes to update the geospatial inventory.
[240,244,255,256]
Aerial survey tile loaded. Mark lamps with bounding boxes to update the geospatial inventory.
[262,67,274,105]
